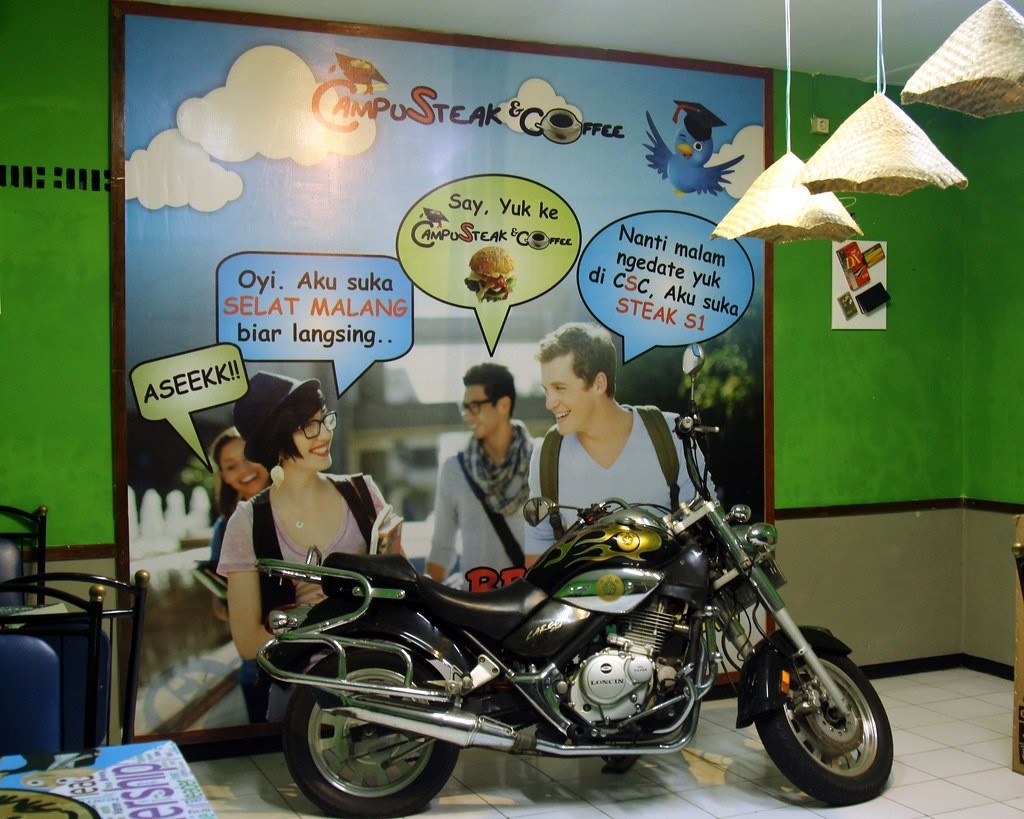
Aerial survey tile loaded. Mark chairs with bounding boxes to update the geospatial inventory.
[0,505,150,756]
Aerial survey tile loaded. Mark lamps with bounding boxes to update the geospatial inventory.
[791,0,970,196]
[900,0,1024,118]
[709,0,864,242]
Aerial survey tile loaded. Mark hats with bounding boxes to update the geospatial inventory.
[233,369,320,444]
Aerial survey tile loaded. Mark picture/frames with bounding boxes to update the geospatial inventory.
[105,0,774,744]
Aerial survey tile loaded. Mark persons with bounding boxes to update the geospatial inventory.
[422,362,540,592]
[206,426,274,618]
[524,320,716,568]
[216,374,409,659]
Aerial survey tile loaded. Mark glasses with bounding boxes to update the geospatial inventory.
[457,398,494,416]
[292,408,338,437]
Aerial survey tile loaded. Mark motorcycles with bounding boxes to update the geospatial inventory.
[252,343,894,819]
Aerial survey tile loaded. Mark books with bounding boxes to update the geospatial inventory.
[370,501,405,557]
[192,559,230,599]
